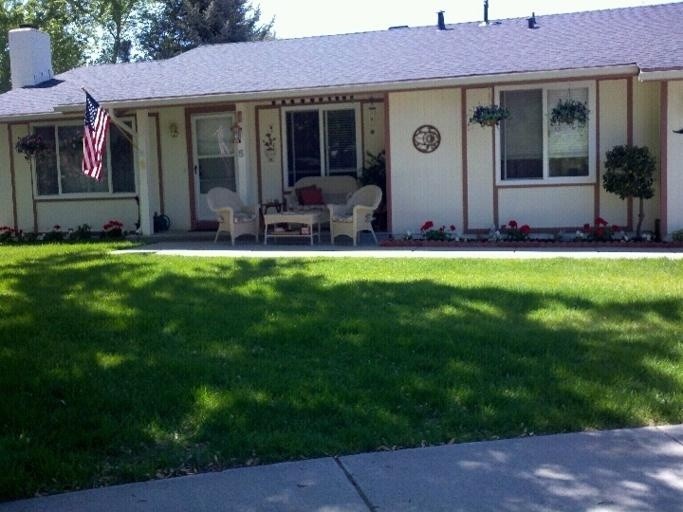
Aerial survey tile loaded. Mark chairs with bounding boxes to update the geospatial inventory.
[206,187,261,247]
[327,184,382,245]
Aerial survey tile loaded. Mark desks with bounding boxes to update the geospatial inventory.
[264,211,321,246]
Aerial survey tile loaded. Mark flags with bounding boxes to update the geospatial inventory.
[81,92,111,182]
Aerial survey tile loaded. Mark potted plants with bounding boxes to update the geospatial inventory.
[468,102,509,128]
[262,124,276,161]
[545,98,591,130]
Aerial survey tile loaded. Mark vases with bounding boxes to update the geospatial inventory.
[70,153,82,169]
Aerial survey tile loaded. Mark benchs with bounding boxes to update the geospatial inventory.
[283,177,357,236]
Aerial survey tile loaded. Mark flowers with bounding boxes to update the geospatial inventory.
[64,130,83,156]
